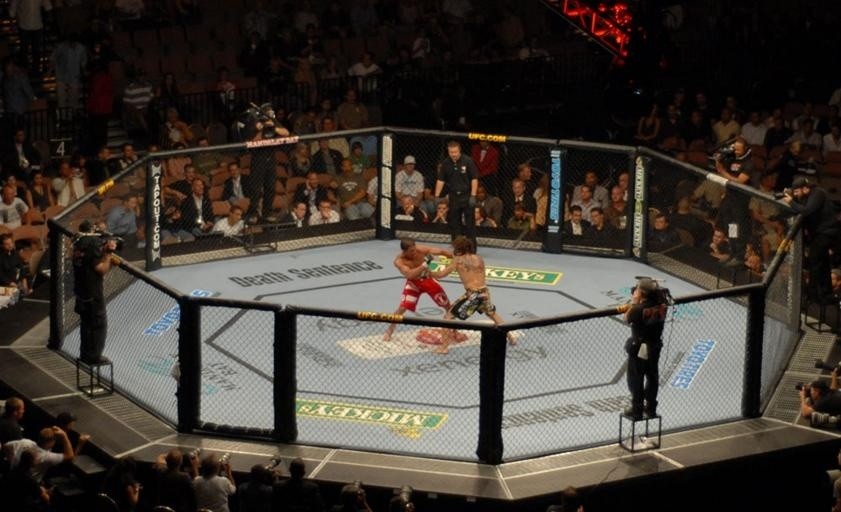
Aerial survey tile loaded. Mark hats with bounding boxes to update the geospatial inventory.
[403,155,415,165]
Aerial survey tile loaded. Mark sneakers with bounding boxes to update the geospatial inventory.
[625,406,644,419]
[644,406,657,418]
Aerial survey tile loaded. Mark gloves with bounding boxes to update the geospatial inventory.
[424,254,433,264]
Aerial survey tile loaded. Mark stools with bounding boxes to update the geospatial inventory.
[619,413,661,451]
[245,221,278,253]
[76,357,112,397]
[804,296,837,332]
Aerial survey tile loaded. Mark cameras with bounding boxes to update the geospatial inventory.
[795,381,811,396]
[774,188,792,199]
[354,478,362,489]
[400,485,413,504]
[814,358,841,376]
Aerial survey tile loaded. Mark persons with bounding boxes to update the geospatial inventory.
[2,2,839,511]
